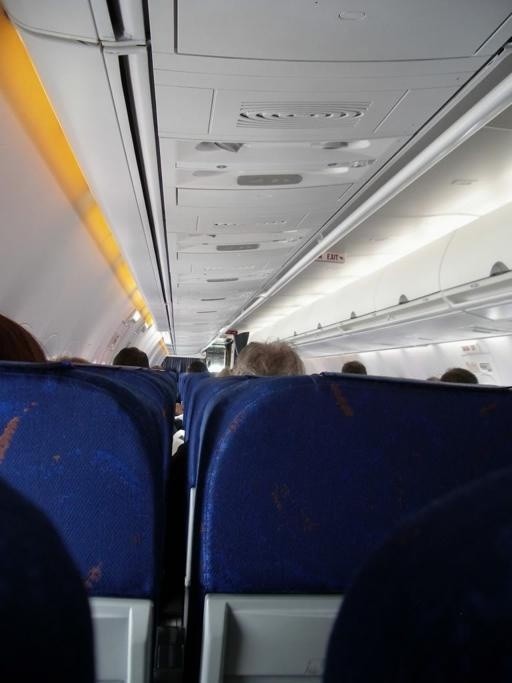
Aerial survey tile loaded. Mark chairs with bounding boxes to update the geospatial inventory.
[1,361,511,682]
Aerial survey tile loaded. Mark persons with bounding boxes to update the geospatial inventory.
[185,360,210,372]
[1,314,45,361]
[229,341,305,377]
[438,366,479,384]
[341,359,370,376]
[112,346,151,368]
